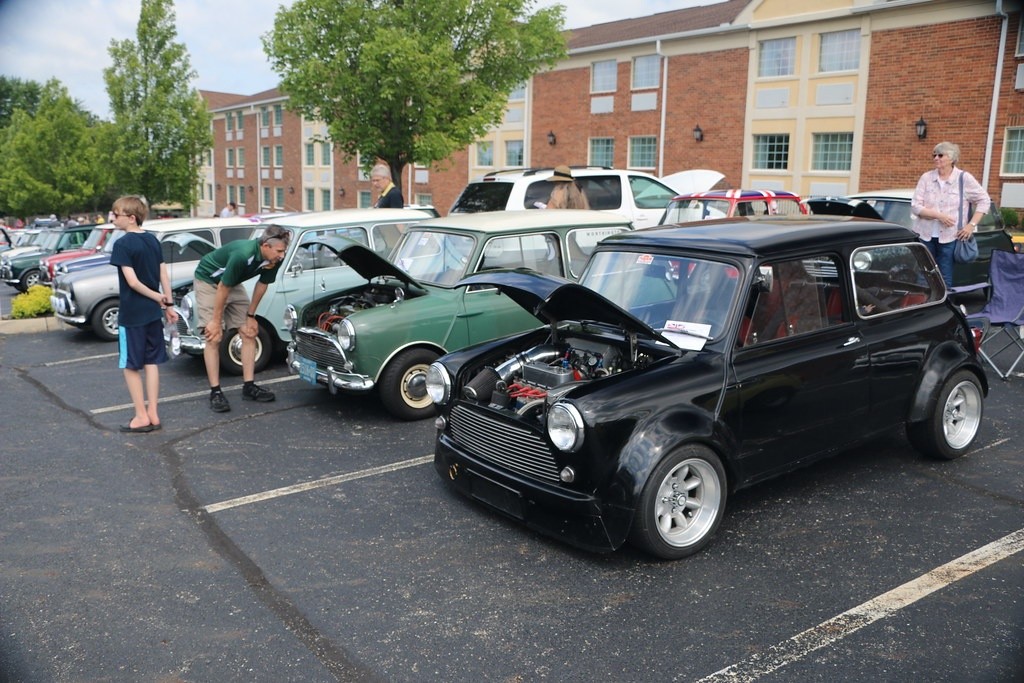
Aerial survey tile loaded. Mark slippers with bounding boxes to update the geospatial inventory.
[149,421,162,430]
[118,418,152,432]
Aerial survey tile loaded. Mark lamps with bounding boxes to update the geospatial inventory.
[248,183,253,193]
[693,124,703,142]
[216,182,221,190]
[339,185,346,198]
[288,185,295,194]
[915,115,927,140]
[547,130,556,146]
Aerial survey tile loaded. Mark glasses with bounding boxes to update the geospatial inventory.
[933,154,948,158]
[264,234,285,243]
[114,212,130,219]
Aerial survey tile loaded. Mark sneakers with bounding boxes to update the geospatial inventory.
[210,392,230,412]
[242,385,275,402]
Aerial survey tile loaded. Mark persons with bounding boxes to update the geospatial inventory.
[371,164,404,232]
[911,141,990,289]
[110,196,178,432]
[67,214,105,225]
[17,218,24,228]
[544,164,591,210]
[194,223,290,412]
[220,201,236,218]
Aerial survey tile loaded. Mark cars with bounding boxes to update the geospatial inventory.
[0,164,1017,566]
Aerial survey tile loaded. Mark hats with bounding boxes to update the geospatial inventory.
[370,164,392,181]
[545,164,576,181]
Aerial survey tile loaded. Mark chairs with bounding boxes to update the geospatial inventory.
[686,266,752,347]
[758,281,843,341]
[947,248,1024,382]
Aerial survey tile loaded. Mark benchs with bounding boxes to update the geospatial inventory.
[772,280,929,318]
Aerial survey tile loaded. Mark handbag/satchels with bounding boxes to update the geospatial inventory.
[954,234,979,265]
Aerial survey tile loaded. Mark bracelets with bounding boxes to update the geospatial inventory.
[165,302,174,306]
[969,221,975,227]
[247,312,254,317]
